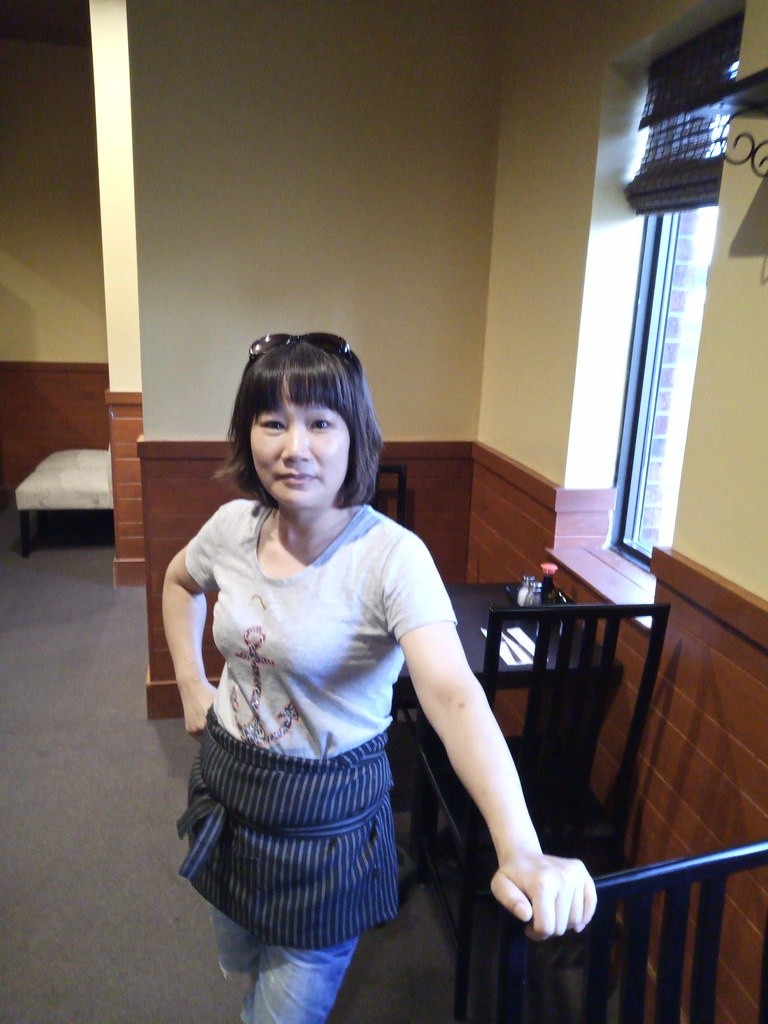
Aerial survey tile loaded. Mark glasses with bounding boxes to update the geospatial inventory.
[249,332,354,364]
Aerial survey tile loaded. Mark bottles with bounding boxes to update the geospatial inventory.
[524,581,542,607]
[517,575,536,607]
[541,563,558,606]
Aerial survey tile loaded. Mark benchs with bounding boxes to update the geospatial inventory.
[14,448,114,560]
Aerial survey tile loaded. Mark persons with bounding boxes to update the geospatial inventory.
[161,333,598,1024]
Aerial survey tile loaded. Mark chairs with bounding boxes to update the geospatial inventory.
[410,599,673,1021]
[497,841,768,1024]
[371,465,407,527]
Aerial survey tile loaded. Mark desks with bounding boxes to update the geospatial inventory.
[392,581,623,707]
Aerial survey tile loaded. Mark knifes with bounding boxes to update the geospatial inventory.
[500,629,534,662]
